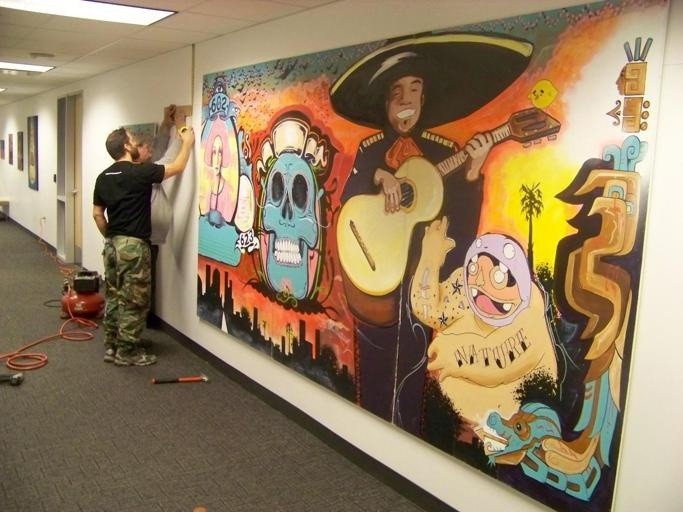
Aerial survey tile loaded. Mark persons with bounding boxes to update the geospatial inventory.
[92,125,196,366]
[338,71,494,436]
[130,102,187,329]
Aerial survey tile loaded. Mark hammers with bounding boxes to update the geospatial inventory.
[150,372,208,384]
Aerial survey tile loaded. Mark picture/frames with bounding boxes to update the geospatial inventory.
[0,116,38,191]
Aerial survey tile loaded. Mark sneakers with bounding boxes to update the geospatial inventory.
[103,337,156,366]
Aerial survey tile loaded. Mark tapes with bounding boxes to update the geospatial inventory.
[181,127,187,132]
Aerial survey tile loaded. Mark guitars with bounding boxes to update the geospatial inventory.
[336,108,561,298]
